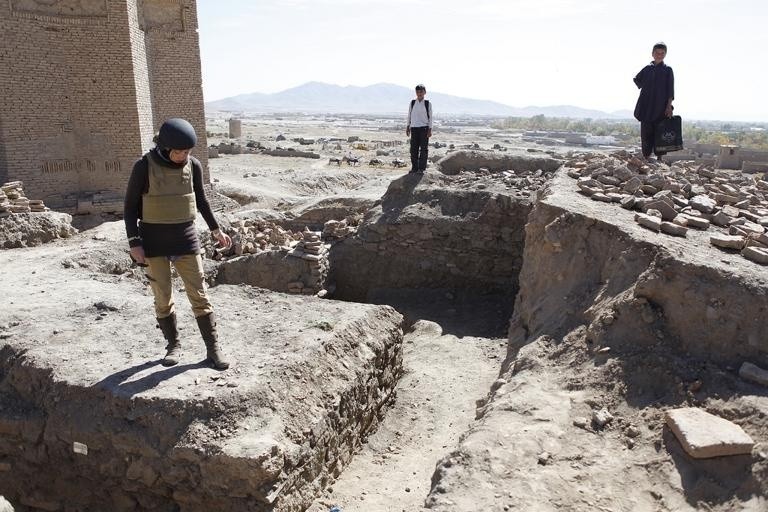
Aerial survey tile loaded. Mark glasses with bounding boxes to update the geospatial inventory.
[130,254,149,267]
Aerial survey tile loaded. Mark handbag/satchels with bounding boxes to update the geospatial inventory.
[654,116,683,152]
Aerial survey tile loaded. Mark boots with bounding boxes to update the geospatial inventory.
[156,311,181,365]
[196,311,230,369]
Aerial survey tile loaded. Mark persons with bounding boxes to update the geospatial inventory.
[633,44,674,160]
[406,85,431,173]
[124,117,231,369]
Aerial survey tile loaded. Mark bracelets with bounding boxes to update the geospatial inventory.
[213,229,221,239]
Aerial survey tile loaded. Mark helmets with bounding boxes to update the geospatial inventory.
[159,118,196,150]
